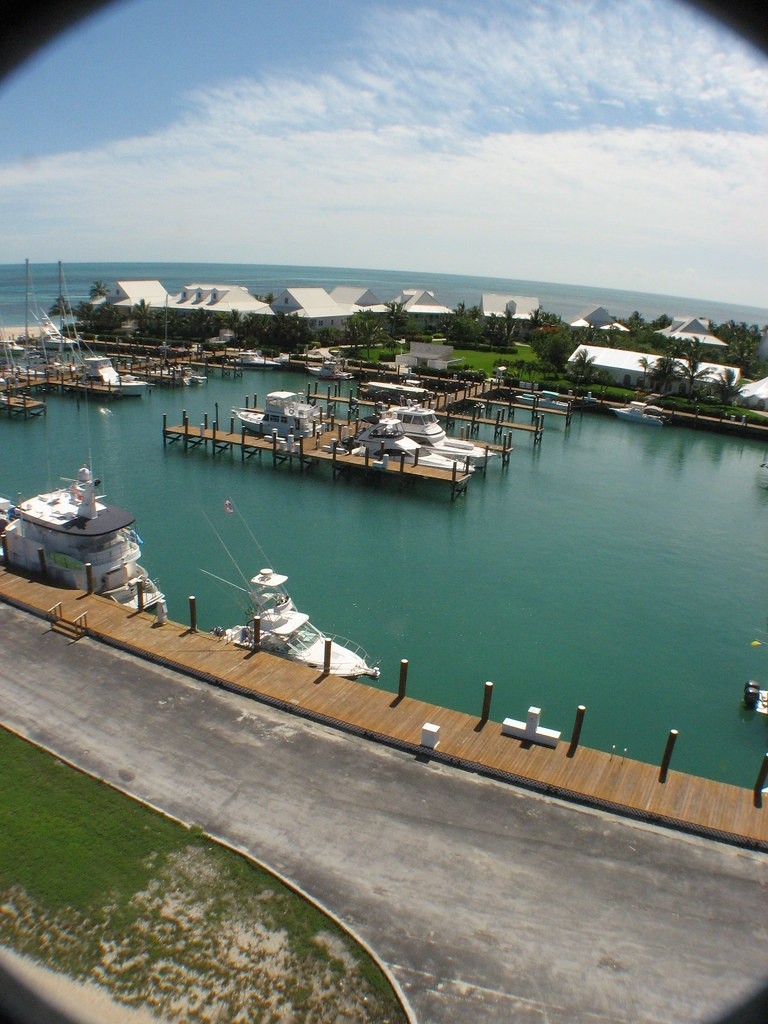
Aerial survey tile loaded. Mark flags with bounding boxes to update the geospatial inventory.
[225,498,233,513]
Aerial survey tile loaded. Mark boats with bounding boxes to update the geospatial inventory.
[610,402,666,427]
[341,413,475,474]
[7,364,44,376]
[227,352,281,370]
[39,322,75,349]
[193,489,382,680]
[1,469,161,624]
[380,399,497,468]
[229,389,322,440]
[306,362,353,380]
[0,341,24,357]
[80,356,147,398]
[148,363,207,385]
[516,394,572,411]
